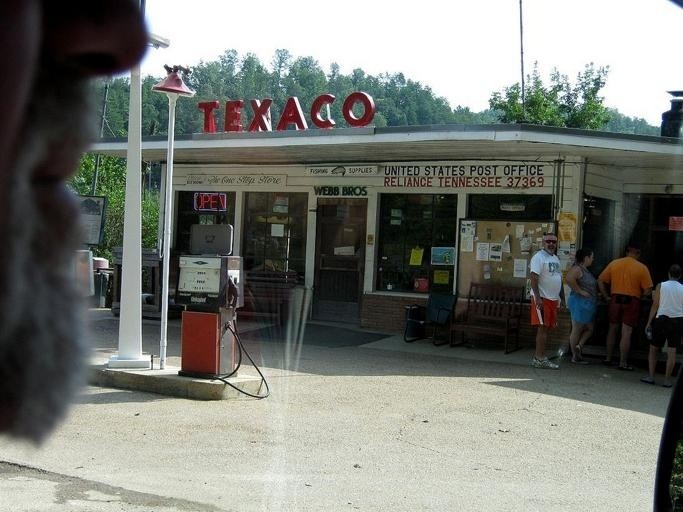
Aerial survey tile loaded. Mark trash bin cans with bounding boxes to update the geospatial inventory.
[90,269,115,308]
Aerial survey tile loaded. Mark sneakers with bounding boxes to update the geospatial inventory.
[531,357,559,369]
[571,345,590,364]
[663,383,672,387]
[640,376,655,384]
[619,365,633,371]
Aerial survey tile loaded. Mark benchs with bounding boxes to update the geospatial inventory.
[449,282,525,354]
[237,271,299,328]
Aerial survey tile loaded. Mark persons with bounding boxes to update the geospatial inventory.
[637,263,682,388]
[527,232,563,369]
[0,0,156,455]
[564,247,599,364]
[596,241,654,372]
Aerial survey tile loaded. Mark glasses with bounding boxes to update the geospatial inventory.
[546,240,557,243]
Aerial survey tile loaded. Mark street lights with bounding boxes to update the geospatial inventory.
[149,64,195,370]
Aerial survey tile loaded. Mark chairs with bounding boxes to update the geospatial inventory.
[404,292,459,347]
